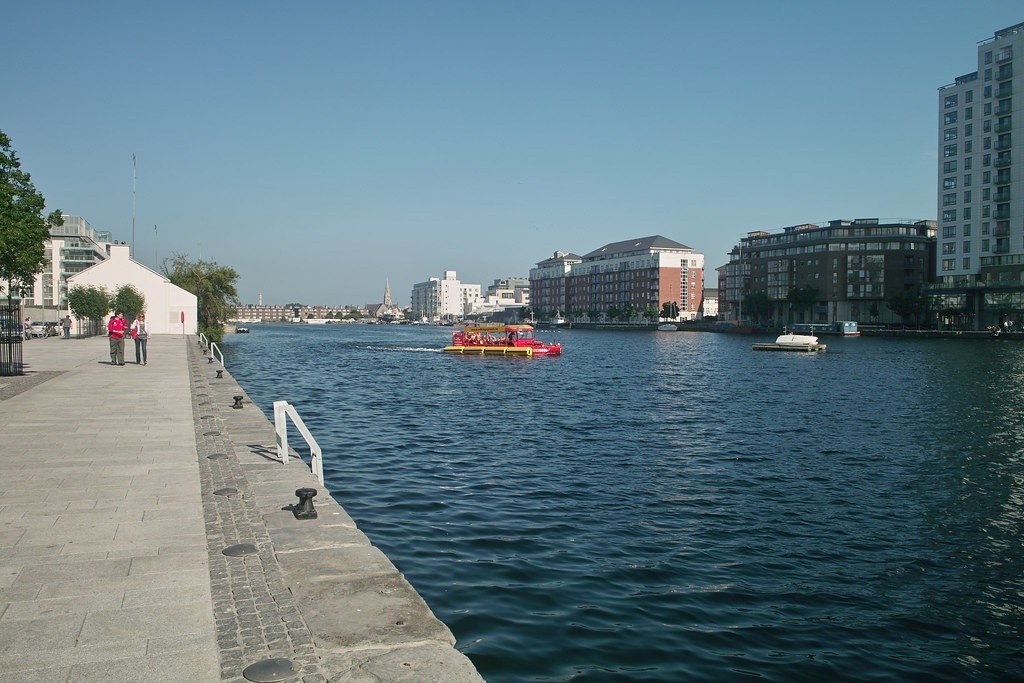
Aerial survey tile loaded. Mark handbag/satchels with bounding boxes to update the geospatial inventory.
[132,322,138,339]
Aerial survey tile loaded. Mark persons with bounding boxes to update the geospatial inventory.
[986,319,1015,332]
[62,315,72,339]
[108,311,128,366]
[459,331,516,345]
[25,316,31,327]
[130,313,151,365]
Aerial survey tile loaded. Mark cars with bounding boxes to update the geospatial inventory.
[0,321,65,343]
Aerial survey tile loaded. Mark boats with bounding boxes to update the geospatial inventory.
[443,321,562,357]
[752,324,827,352]
[785,320,861,337]
[657,323,678,332]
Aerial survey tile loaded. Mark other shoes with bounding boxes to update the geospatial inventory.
[144,361,147,366]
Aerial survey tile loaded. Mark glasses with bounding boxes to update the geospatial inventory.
[139,316,144,318]
